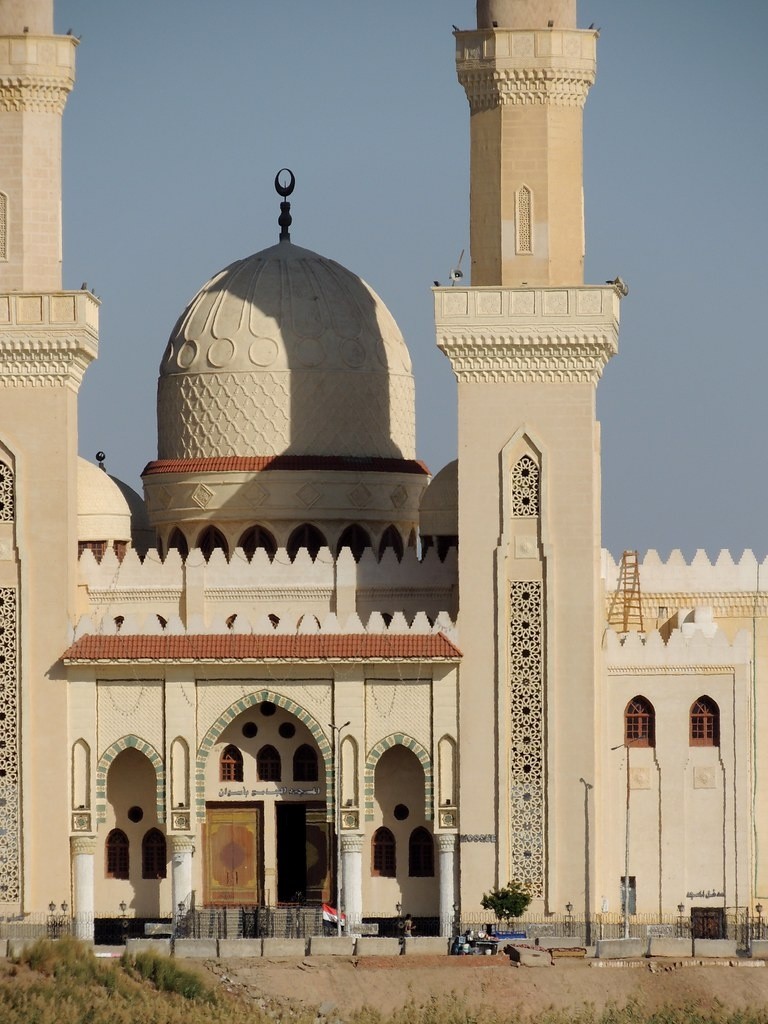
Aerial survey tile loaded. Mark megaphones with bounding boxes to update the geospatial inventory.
[615,276,629,296]
[450,269,463,282]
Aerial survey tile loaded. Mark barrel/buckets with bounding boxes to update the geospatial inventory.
[485,949,491,955]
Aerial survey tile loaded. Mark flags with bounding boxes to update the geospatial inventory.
[321,903,346,931]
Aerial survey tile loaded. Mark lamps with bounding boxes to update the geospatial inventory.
[178,901,185,913]
[49,901,57,912]
[396,901,402,914]
[119,900,128,912]
[677,901,684,913]
[564,901,573,914]
[453,902,459,911]
[61,900,68,914]
[756,903,763,913]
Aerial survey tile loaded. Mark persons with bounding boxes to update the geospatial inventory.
[402,914,416,937]
